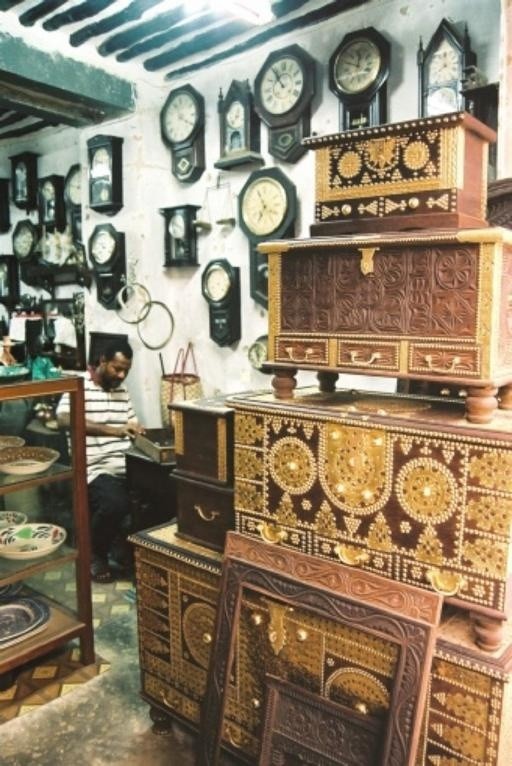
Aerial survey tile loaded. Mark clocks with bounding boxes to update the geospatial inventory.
[85,135,124,214]
[416,19,476,118]
[255,42,317,162]
[239,169,298,308]
[161,204,201,268]
[214,79,266,170]
[328,26,392,131]
[160,86,206,182]
[87,223,125,309]
[1,137,85,345]
[200,260,240,348]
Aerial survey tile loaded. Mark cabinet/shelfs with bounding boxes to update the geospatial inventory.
[0,373,94,675]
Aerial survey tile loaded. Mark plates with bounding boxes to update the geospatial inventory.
[0,365,30,382]
[0,580,52,650]
[0,435,61,475]
[0,511,68,560]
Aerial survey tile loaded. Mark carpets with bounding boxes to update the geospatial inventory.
[0,642,112,724]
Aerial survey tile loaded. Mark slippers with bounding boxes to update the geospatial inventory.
[89,562,115,583]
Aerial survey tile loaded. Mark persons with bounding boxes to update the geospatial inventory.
[57,341,177,582]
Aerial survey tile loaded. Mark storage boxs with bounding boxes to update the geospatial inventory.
[172,465,233,555]
[300,110,500,236]
[257,226,512,427]
[168,391,280,481]
[134,520,511,765]
[229,385,512,652]
